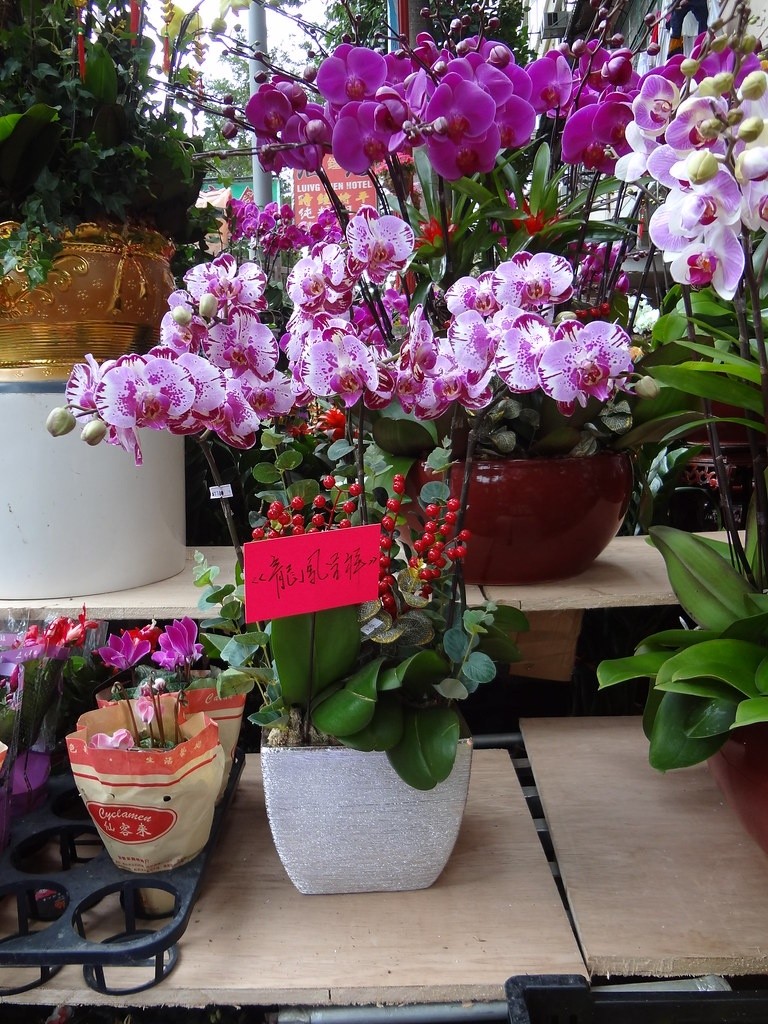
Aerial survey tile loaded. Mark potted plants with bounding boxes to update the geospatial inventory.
[0,1,226,371]
[588,482,768,859]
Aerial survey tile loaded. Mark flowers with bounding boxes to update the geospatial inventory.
[187,475,527,790]
[40,1,766,464]
[0,607,202,857]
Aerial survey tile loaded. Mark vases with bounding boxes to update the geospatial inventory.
[260,703,475,896]
[692,398,748,442]
[388,451,636,584]
[64,676,246,874]
